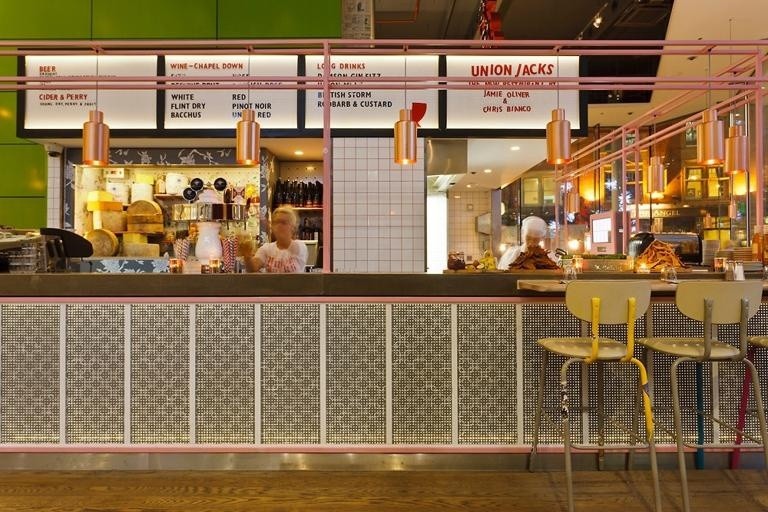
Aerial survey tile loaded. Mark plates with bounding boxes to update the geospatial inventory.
[744,260,764,273]
[718,247,753,261]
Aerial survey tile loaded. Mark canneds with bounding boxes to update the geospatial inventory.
[201,265,210,274]
[209,267,216,273]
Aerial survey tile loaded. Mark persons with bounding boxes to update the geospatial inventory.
[496,214,558,269]
[236,203,307,274]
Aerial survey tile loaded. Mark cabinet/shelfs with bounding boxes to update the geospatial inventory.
[273,206,323,273]
[153,193,260,244]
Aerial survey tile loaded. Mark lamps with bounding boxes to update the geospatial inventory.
[237,49,260,166]
[566,165,581,214]
[83,49,110,168]
[695,49,724,167]
[724,19,747,176]
[647,114,665,195]
[395,47,417,165]
[593,14,603,29]
[546,49,572,165]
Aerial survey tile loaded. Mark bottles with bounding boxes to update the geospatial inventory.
[660,265,678,283]
[724,260,744,281]
[274,177,320,208]
[301,218,320,240]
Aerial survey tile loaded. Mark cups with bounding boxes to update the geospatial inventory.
[209,259,222,274]
[714,257,728,273]
[571,258,582,274]
[237,231,255,258]
[232,203,241,221]
[170,259,182,274]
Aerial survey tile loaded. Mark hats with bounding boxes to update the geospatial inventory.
[523,216,548,239]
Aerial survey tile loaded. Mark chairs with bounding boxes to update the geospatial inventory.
[625,279,767,512]
[728,336,768,470]
[528,279,663,512]
